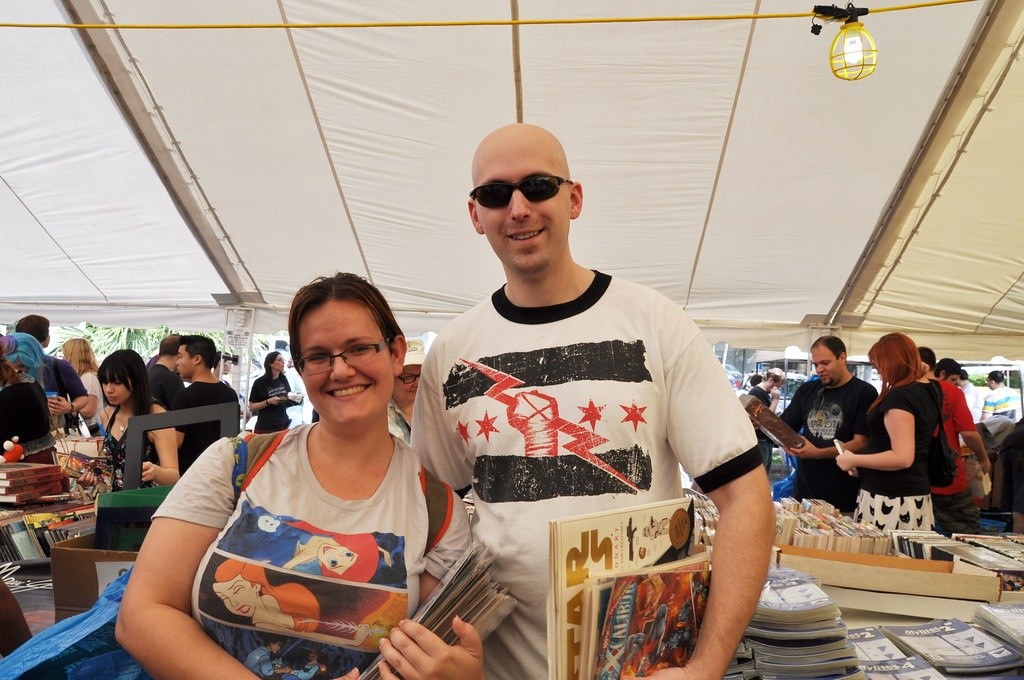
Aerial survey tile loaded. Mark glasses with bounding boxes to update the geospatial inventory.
[276,359,286,362]
[297,337,391,374]
[398,374,420,384]
[470,176,573,208]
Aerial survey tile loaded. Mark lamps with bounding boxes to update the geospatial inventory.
[813,0,876,80]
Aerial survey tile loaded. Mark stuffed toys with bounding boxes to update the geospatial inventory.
[0,436,25,463]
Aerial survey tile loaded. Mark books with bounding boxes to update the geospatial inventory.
[0,436,113,564]
[739,394,804,451]
[543,495,1024,680]
[833,439,858,477]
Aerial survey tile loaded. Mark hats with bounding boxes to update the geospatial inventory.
[403,339,427,366]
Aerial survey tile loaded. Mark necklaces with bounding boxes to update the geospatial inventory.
[120,413,127,433]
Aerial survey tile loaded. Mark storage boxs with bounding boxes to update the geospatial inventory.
[739,394,805,451]
[51,533,140,624]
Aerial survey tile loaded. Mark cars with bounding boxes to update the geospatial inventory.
[725,364,743,389]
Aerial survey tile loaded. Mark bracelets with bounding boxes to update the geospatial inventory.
[266,399,269,406]
[70,403,74,412]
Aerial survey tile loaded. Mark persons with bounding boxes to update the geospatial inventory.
[749,333,1024,538]
[387,337,425,445]
[77,350,180,490]
[249,351,291,434]
[0,315,106,454]
[115,273,485,680]
[143,333,252,477]
[409,123,778,680]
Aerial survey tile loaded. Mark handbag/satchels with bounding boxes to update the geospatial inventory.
[929,380,955,488]
[51,358,92,436]
